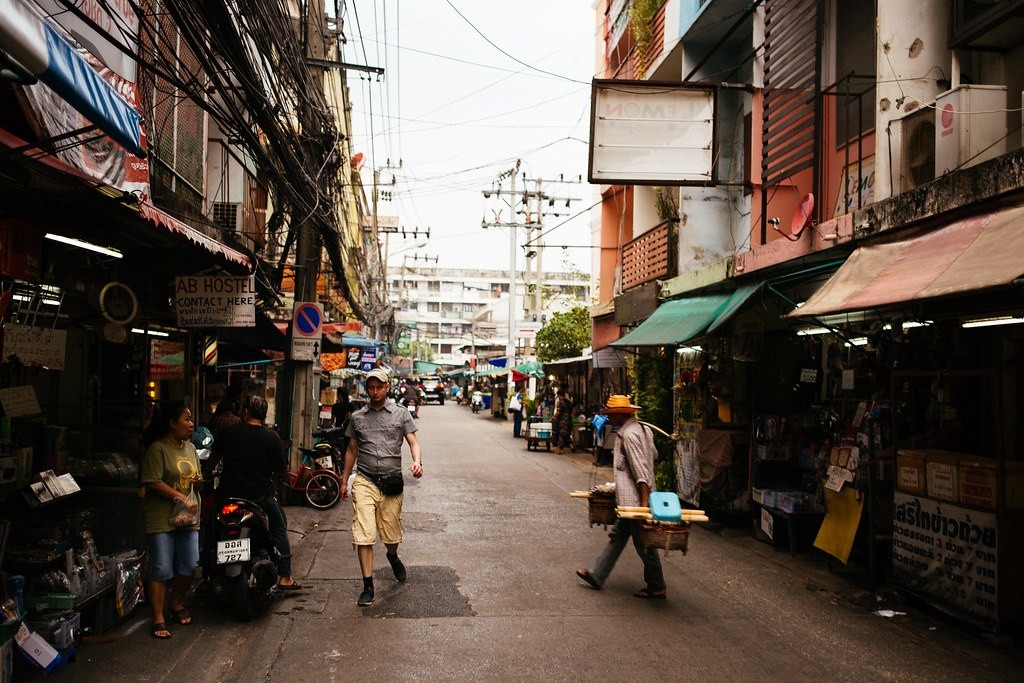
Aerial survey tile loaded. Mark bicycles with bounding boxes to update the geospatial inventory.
[472,402,481,414]
[284,447,342,511]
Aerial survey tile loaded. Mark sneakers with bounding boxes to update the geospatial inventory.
[386,551,406,582]
[359,586,375,605]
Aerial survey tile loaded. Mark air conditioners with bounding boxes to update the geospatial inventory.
[209,200,243,233]
[898,100,938,192]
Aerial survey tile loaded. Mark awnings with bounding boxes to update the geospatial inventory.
[787,204,1024,346]
[606,281,770,362]
[0,127,254,270]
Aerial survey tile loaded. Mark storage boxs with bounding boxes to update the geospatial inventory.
[896,449,1003,510]
[26,611,81,649]
[592,424,617,450]
[753,487,814,512]
[321,388,337,405]
[0,639,14,683]
[27,591,75,608]
[530,422,553,437]
[21,631,62,672]
[82,593,131,632]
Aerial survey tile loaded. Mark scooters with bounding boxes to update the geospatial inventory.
[204,493,294,621]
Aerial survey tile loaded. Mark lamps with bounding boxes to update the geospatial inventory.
[883,319,933,330]
[796,326,838,336]
[844,337,868,347]
[44,232,123,260]
[960,309,1024,328]
[522,245,537,261]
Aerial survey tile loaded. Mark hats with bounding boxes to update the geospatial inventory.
[364,369,389,383]
[598,395,642,414]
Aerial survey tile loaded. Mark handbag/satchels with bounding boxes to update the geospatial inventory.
[509,392,522,411]
[170,484,200,527]
[356,466,404,496]
[522,406,527,418]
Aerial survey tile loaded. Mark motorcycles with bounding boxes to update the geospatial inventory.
[312,426,346,477]
[406,400,417,419]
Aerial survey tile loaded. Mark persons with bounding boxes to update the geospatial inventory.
[471,387,482,414]
[331,387,355,427]
[576,395,668,597]
[396,378,426,413]
[513,385,528,438]
[463,380,468,399]
[551,383,575,453]
[140,398,204,639]
[204,395,304,589]
[339,368,423,607]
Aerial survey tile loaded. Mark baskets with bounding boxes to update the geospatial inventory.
[642,529,690,557]
[27,609,81,649]
[589,504,615,530]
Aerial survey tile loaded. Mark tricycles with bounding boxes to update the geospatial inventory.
[522,416,556,451]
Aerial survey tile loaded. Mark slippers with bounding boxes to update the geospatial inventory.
[576,568,603,590]
[153,617,172,638]
[278,582,303,589]
[168,603,193,624]
[633,587,668,600]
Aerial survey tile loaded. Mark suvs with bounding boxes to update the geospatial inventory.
[417,376,444,405]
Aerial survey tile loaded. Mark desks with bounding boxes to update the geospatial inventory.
[751,503,825,557]
[890,490,1000,625]
